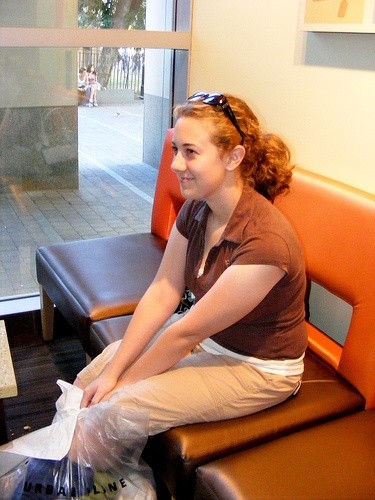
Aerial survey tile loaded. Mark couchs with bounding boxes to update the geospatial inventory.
[36,127,375,500]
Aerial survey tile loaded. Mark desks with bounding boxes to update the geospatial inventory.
[0,319,18,446]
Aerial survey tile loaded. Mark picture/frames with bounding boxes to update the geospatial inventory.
[299,0,375,33]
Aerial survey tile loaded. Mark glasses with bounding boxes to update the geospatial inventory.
[186,91,244,147]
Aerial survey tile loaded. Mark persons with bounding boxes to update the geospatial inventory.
[78,66,100,107]
[65,92,307,472]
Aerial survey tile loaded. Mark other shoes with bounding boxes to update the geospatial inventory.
[93,103,98,107]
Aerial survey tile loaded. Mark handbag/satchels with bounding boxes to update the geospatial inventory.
[0,379,157,500]
